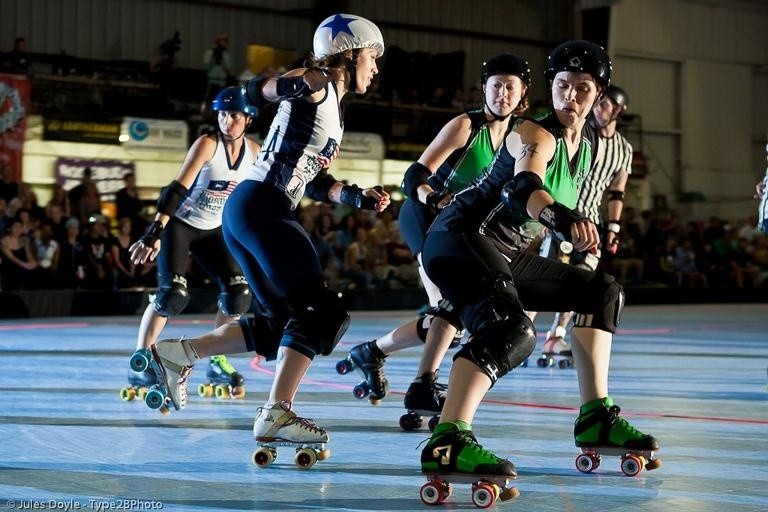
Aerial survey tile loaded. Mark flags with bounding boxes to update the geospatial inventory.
[0,76,33,183]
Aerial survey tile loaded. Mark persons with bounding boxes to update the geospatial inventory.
[335,54,534,434]
[127,14,392,469]
[517,86,635,371]
[419,39,662,507]
[120,85,265,408]
[1,168,767,294]
[751,144,767,237]
[202,33,239,78]
[343,76,484,111]
[5,36,34,72]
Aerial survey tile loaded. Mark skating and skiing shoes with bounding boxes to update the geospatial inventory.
[399,373,446,432]
[249,400,330,470]
[536,327,573,367]
[121,338,246,415]
[574,397,661,477]
[422,425,519,507]
[333,341,387,406]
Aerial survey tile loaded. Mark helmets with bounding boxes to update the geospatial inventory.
[213,86,260,116]
[605,86,629,111]
[543,40,612,84]
[481,53,531,89]
[313,13,386,60]
[88,215,109,223]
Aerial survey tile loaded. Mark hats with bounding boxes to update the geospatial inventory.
[65,218,81,229]
